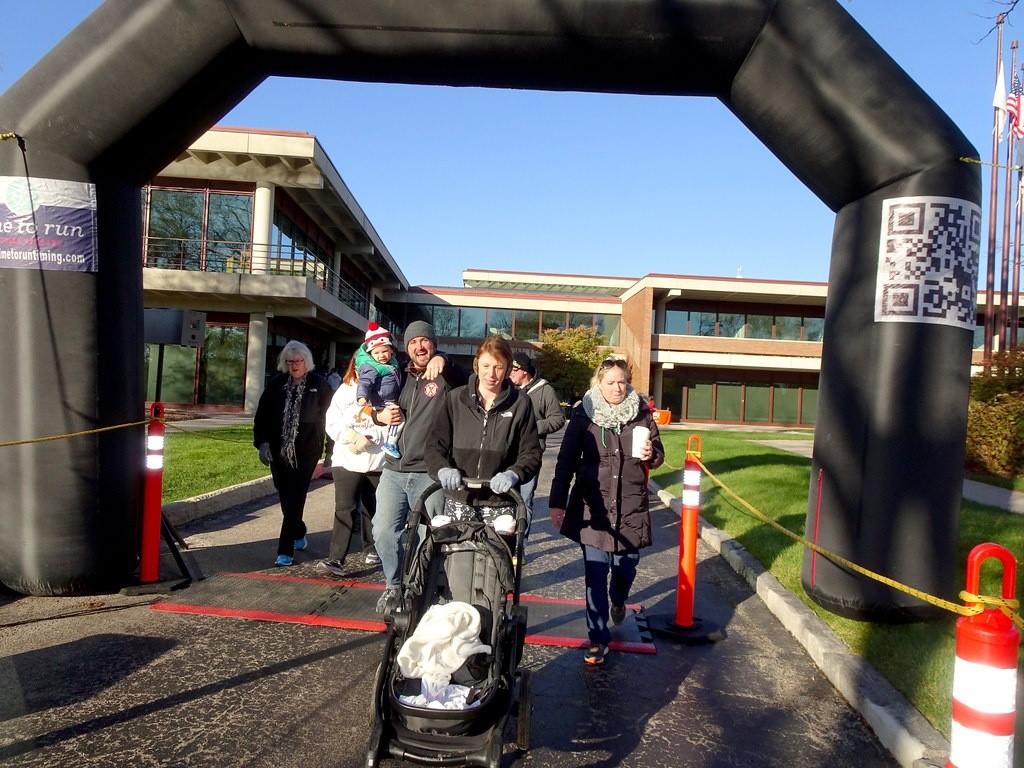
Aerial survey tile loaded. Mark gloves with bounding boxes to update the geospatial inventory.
[490,469,518,494]
[347,430,373,454]
[322,453,334,467]
[438,467,465,491]
[258,442,273,466]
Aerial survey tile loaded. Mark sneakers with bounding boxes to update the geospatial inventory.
[583,642,609,664]
[381,442,401,458]
[275,555,293,565]
[365,552,381,564]
[322,560,344,576]
[610,603,626,625]
[294,535,307,549]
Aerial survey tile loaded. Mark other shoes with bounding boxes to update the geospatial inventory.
[512,548,527,565]
[376,587,402,613]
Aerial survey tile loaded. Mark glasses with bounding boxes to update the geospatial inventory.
[285,358,304,365]
[599,359,627,372]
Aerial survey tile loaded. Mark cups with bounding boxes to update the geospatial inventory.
[632,429,650,458]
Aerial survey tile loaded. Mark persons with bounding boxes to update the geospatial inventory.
[315,348,383,576]
[353,321,414,460]
[571,390,582,419]
[371,320,473,615]
[508,352,566,566]
[422,336,543,557]
[549,359,665,666]
[472,356,478,374]
[639,392,656,416]
[252,340,337,567]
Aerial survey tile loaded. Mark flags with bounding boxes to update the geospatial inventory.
[982,52,1024,146]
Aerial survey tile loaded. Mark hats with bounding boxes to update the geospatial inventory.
[403,321,437,352]
[511,351,536,378]
[365,321,395,352]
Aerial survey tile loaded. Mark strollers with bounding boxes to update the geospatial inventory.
[364,477,530,768]
